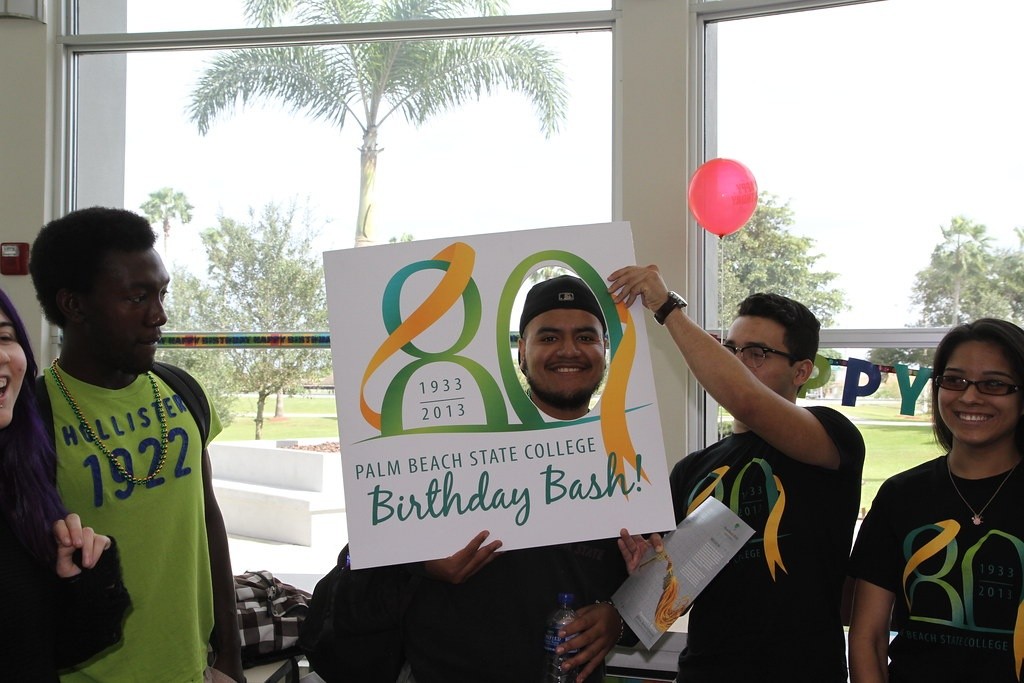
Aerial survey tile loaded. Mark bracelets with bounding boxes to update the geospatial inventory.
[596,599,625,643]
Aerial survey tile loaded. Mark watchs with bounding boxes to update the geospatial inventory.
[654,291,687,325]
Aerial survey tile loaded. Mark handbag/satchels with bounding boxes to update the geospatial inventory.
[233,570,315,655]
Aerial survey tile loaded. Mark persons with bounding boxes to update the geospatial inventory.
[607,264,866,683]
[28,206,245,683]
[847,319,1024,683]
[0,289,132,683]
[300,275,641,683]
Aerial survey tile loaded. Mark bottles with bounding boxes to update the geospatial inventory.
[541,590,581,683]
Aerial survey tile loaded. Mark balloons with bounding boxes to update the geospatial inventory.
[688,158,759,240]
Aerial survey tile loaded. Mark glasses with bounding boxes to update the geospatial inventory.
[933,375,1024,396]
[722,344,797,368]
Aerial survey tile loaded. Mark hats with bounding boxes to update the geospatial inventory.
[519,275,608,339]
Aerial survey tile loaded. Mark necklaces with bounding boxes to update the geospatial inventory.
[948,454,1019,525]
[50,358,168,485]
